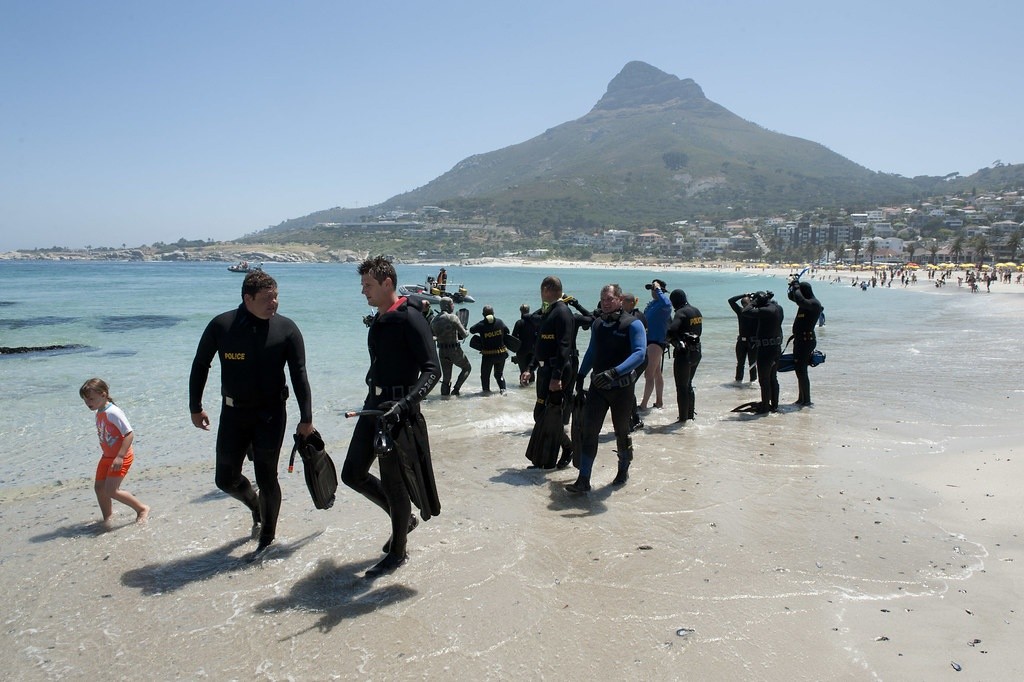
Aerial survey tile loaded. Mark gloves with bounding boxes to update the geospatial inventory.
[592,369,615,389]
[577,374,585,392]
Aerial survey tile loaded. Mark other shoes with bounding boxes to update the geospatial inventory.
[557,443,575,468]
[366,554,409,580]
[613,464,629,485]
[382,515,420,554]
[566,478,590,492]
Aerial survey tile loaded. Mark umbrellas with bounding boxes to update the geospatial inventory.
[756,262,1024,272]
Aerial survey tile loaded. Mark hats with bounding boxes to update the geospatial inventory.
[645,279,670,296]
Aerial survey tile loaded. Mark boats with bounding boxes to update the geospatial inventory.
[228,265,257,272]
[426,274,476,303]
[399,283,442,304]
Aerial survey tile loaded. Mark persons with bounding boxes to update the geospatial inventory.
[621,293,649,433]
[437,267,447,292]
[728,289,784,414]
[431,297,472,395]
[421,299,435,323]
[697,266,1022,294]
[564,283,647,493]
[470,304,510,395]
[670,289,703,424]
[787,276,821,407]
[341,257,442,576]
[80,378,150,530]
[189,270,312,561]
[520,276,574,470]
[637,279,672,412]
[562,293,596,424]
[237,262,265,269]
[515,304,535,386]
